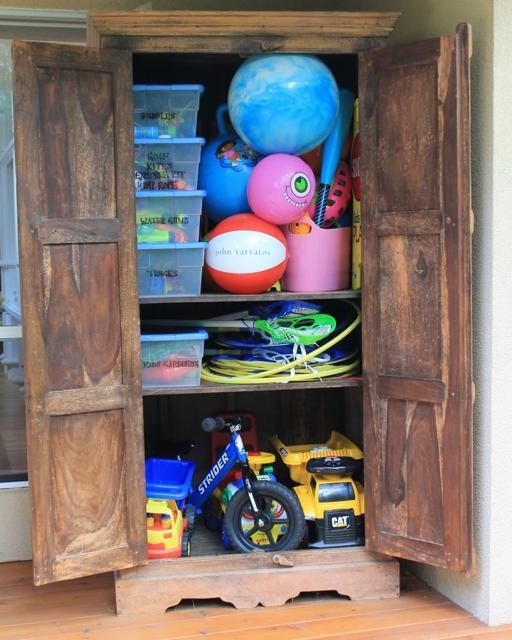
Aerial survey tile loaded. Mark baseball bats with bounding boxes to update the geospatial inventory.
[314,87,357,229]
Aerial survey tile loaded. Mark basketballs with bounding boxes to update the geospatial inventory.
[202,213,290,293]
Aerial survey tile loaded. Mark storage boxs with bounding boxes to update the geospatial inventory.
[129,80,209,389]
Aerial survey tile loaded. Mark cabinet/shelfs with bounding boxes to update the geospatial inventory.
[10,5,476,624]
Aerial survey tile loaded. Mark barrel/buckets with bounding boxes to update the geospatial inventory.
[280,217,352,291]
